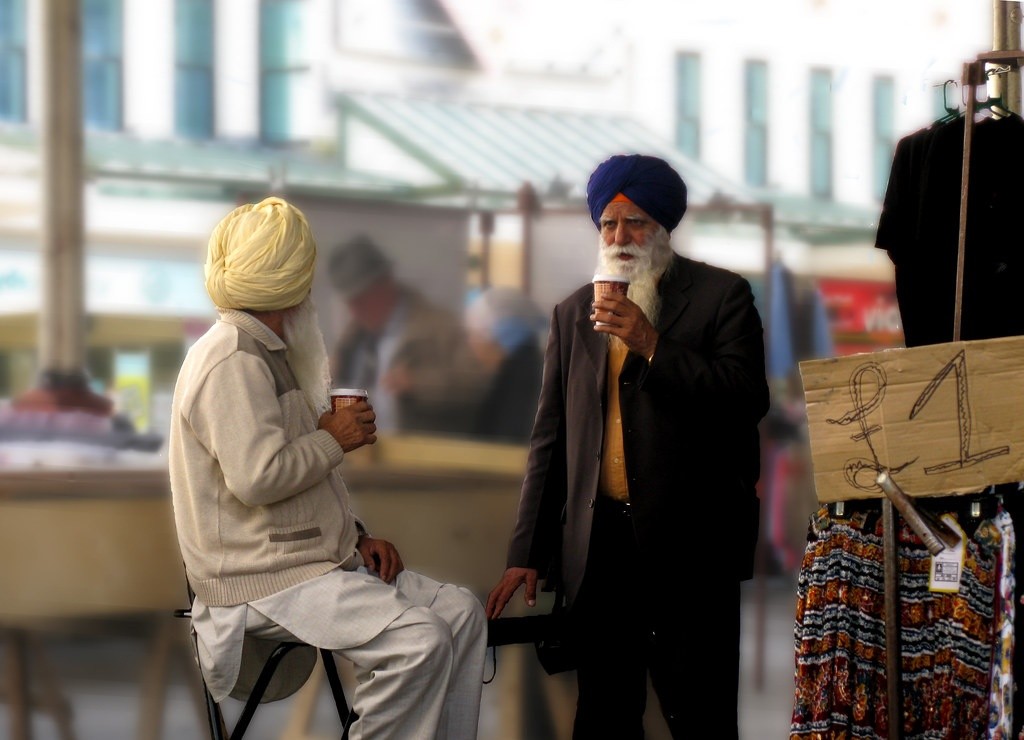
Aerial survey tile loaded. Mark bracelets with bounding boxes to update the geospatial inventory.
[649,354,654,366]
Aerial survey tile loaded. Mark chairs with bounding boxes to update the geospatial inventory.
[173,562,360,740]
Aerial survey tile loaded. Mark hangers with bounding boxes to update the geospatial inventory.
[925,68,1011,131]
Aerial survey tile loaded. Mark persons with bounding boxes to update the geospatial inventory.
[328,241,459,429]
[484,156,769,740]
[389,283,548,443]
[167,197,488,740]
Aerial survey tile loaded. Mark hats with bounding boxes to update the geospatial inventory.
[205,197,314,310]
[585,153,687,233]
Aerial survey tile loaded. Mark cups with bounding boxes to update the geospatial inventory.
[328,388,368,415]
[592,273,630,327]
